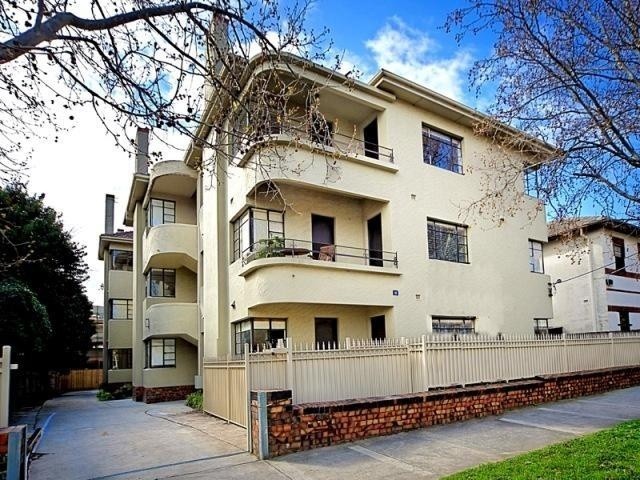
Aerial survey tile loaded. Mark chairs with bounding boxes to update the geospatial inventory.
[309,246,334,261]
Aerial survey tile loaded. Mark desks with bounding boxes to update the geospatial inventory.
[272,247,309,259]
